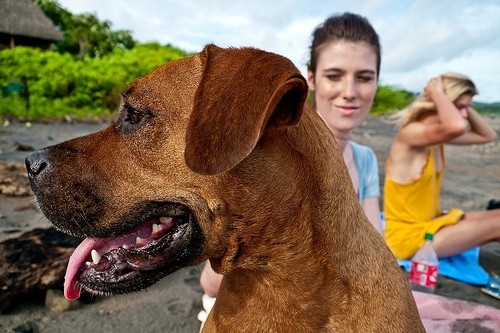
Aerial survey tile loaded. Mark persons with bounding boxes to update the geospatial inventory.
[383,71,500,258]
[197,12,382,333]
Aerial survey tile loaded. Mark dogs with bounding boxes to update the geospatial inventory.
[25,43,427,333]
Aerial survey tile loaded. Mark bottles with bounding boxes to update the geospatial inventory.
[408,233,438,294]
